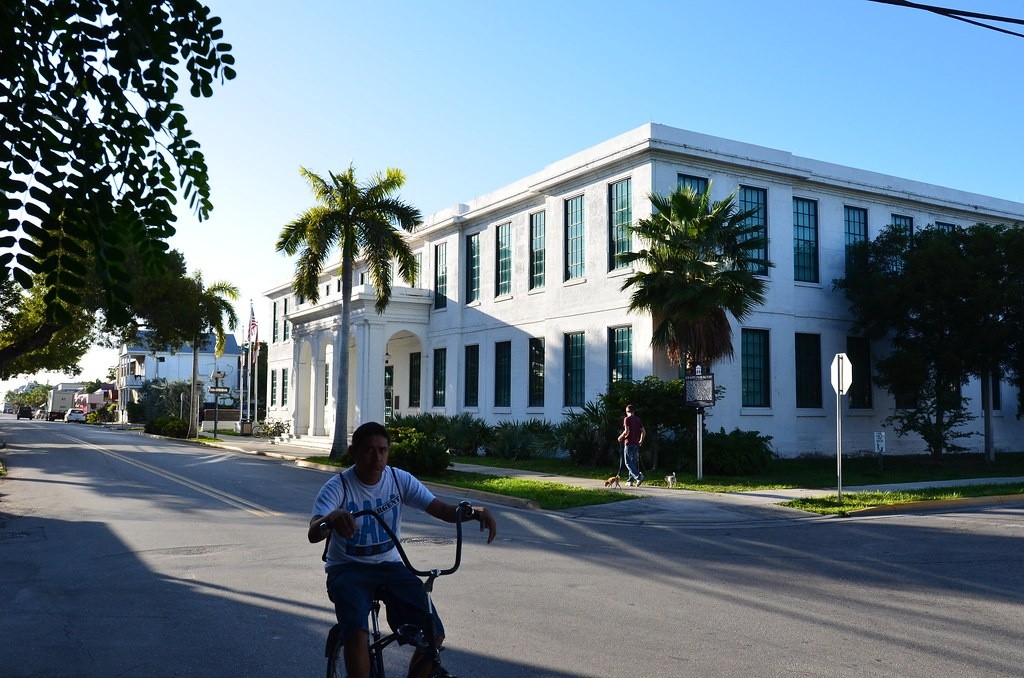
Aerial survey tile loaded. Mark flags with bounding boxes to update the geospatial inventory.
[240,307,260,366]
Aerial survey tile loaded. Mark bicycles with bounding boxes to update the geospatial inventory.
[252,420,290,439]
[320,502,479,678]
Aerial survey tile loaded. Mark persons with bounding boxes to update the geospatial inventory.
[618,405,646,487]
[309,422,496,678]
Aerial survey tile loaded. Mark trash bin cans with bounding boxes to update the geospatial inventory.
[240,418,253,435]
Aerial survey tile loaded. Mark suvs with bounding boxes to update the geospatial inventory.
[17,407,33,420]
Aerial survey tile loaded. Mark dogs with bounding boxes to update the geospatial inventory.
[664,472,676,489]
[604,475,621,489]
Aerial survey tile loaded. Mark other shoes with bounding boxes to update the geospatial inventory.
[636,479,644,487]
[629,481,634,486]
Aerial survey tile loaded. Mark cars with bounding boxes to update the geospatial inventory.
[64,408,86,424]
[35,409,47,419]
[41,412,48,421]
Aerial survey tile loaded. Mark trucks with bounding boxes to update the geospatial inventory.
[47,390,75,421]
